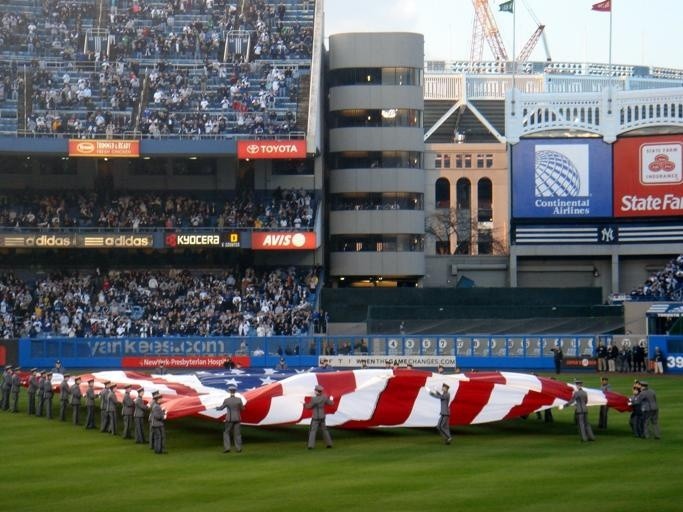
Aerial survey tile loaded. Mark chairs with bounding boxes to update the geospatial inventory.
[0,0,321,356]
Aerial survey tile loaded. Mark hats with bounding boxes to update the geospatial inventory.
[314,384,325,392]
[574,380,583,385]
[228,385,238,392]
[639,380,649,386]
[442,383,449,389]
[4,358,164,401]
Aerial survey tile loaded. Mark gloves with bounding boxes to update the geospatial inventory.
[329,393,333,401]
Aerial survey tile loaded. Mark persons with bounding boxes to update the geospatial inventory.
[629,253,683,300]
[428,384,454,444]
[1,161,313,233]
[392,361,401,369]
[0,1,314,139]
[436,364,446,374]
[558,380,596,443]
[1,264,368,375]
[599,377,610,430]
[593,335,665,376]
[0,365,169,456]
[300,384,335,450]
[405,363,415,370]
[543,409,556,424]
[215,387,243,454]
[384,360,392,369]
[548,344,568,374]
[625,375,662,440]
[453,367,460,374]
[536,411,543,420]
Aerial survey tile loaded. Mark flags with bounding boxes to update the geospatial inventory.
[590,0,613,13]
[497,1,517,14]
[10,366,637,430]
